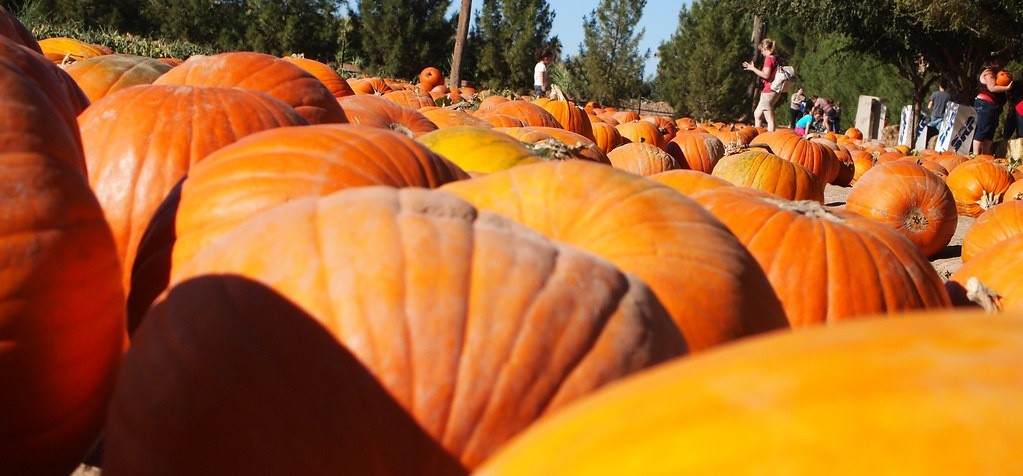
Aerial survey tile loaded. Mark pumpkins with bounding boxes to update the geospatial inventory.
[0,4,1023,476]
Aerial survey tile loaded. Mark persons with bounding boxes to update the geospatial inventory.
[972,55,1014,156]
[790,86,806,130]
[533,48,554,100]
[1001,74,1023,141]
[927,79,950,132]
[793,106,824,136]
[804,93,841,134]
[742,38,784,132]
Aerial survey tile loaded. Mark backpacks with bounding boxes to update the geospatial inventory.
[770,65,795,93]
